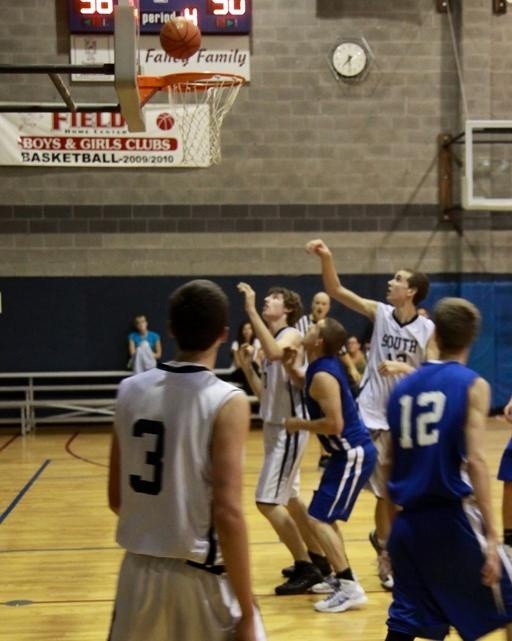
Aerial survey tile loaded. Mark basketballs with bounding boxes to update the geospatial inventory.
[160,17,203,58]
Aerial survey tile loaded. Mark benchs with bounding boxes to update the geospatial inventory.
[0,368,264,435]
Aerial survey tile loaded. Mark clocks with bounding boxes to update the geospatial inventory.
[327,32,376,82]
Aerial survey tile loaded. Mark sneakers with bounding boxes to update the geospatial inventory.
[276,531,391,612]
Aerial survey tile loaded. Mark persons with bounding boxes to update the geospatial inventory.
[304,235,439,594]
[278,317,374,616]
[369,296,511,641]
[125,289,374,413]
[103,278,268,641]
[232,276,332,596]
[495,397,512,549]
